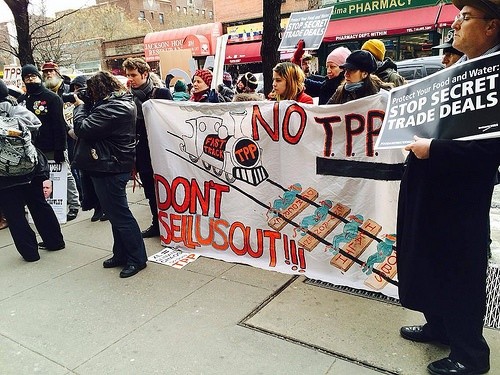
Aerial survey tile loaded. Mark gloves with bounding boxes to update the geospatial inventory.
[208,89,219,103]
[53,150,64,163]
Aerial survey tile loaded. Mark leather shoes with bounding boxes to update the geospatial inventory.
[400,326,449,347]
[0,216,8,229]
[427,357,490,375]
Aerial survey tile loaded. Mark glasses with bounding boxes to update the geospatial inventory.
[454,13,485,22]
[23,74,37,80]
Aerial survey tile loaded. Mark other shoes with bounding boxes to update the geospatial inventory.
[140,226,160,237]
[67,209,78,219]
[38,242,49,248]
[91,213,107,221]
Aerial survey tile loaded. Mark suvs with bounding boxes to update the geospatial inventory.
[397,55,446,84]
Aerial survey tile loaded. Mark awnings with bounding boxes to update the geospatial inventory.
[437,4,461,29]
[143,23,222,64]
[225,39,262,65]
[322,4,441,42]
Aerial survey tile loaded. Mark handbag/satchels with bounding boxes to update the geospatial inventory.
[32,148,50,182]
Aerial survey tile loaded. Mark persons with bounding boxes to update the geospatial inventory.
[73,72,148,278]
[43,180,52,199]
[0,77,65,262]
[432,29,464,68]
[0,39,407,238]
[396,0,500,375]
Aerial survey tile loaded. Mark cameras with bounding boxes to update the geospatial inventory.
[62,89,92,106]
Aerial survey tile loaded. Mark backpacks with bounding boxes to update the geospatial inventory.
[0,105,39,177]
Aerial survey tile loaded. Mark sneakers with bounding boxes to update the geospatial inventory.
[103,255,122,267]
[120,262,146,278]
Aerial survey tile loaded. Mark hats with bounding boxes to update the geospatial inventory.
[431,29,464,56]
[326,39,385,74]
[21,65,42,82]
[192,69,213,87]
[450,0,500,20]
[290,52,312,60]
[242,72,258,89]
[40,61,59,71]
[222,72,232,83]
[174,80,186,92]
